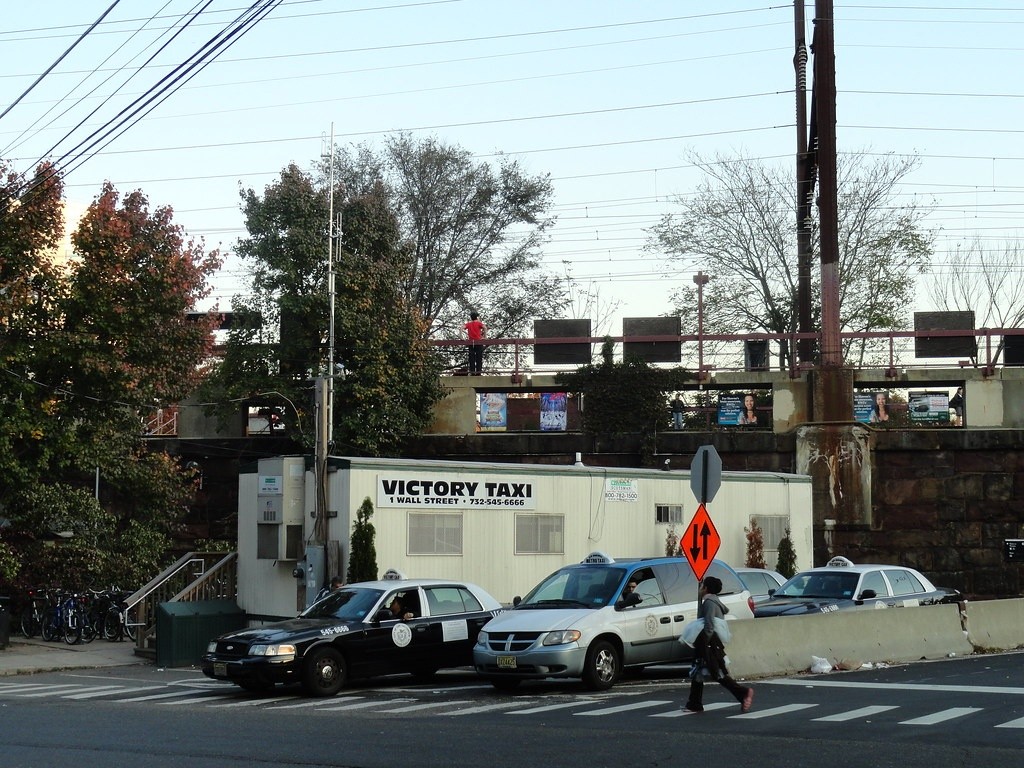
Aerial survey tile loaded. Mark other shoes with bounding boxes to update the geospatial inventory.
[741,687,753,712]
[682,707,704,714]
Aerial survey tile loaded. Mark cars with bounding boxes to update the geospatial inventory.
[751,555,949,621]
[200,569,505,697]
[735,567,802,606]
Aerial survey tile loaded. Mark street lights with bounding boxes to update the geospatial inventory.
[692,270,710,379]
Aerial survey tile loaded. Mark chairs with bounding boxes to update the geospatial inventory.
[820,575,844,597]
[583,584,611,605]
[378,611,392,619]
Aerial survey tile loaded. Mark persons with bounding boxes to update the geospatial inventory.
[625,578,637,593]
[465,313,487,376]
[869,392,889,422]
[670,393,685,429]
[390,596,417,619]
[738,394,758,424]
[314,576,343,602]
[951,388,962,426]
[680,577,754,711]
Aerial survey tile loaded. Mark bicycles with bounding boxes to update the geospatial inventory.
[19,585,143,647]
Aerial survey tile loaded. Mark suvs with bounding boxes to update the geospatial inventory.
[472,550,753,691]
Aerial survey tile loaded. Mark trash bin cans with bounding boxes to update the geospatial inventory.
[154,599,246,668]
